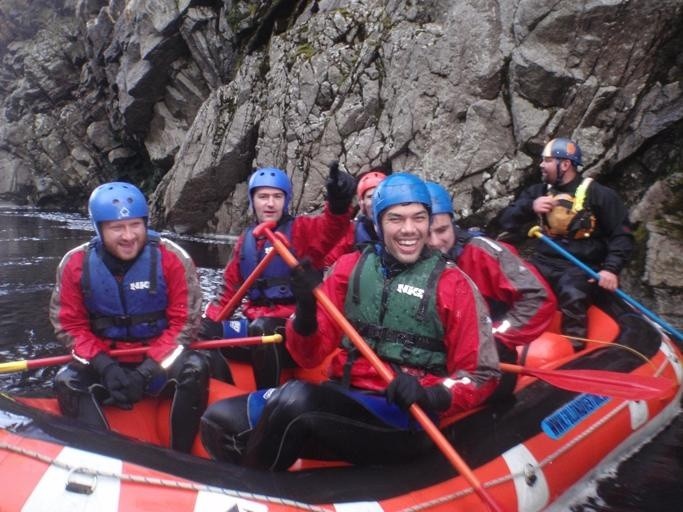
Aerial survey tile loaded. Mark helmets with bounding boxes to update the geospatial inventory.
[87,181,149,243]
[248,167,292,215]
[370,172,432,241]
[424,180,453,214]
[541,137,582,162]
[357,171,387,214]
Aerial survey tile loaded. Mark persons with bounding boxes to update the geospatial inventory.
[199,170,503,476]
[198,158,358,390]
[323,170,388,278]
[422,181,558,440]
[47,178,210,453]
[493,137,635,352]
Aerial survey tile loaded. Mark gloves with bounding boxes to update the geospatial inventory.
[387,372,429,412]
[104,365,128,403]
[289,256,324,304]
[119,371,146,409]
[321,160,358,218]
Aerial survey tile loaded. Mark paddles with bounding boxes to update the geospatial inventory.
[500,363,674,400]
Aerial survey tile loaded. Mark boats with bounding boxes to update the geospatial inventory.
[0,284,682,512]
